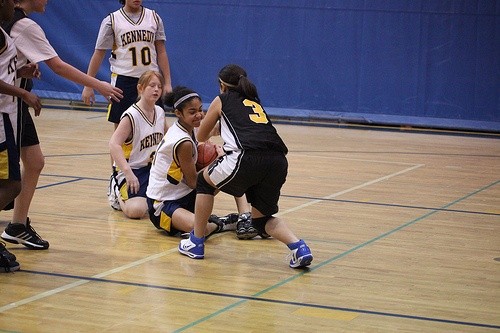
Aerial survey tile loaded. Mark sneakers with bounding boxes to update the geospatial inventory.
[236,212,272,239]
[208,213,239,232]
[178,231,205,259]
[1,217,49,249]
[0,241,20,274]
[108,175,122,210]
[287,239,313,267]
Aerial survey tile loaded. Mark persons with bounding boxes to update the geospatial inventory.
[0,0,123,273]
[108,71,169,218]
[81,0,173,133]
[145,86,260,240]
[178,65,314,269]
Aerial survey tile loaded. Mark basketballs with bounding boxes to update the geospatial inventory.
[195,143,218,169]
